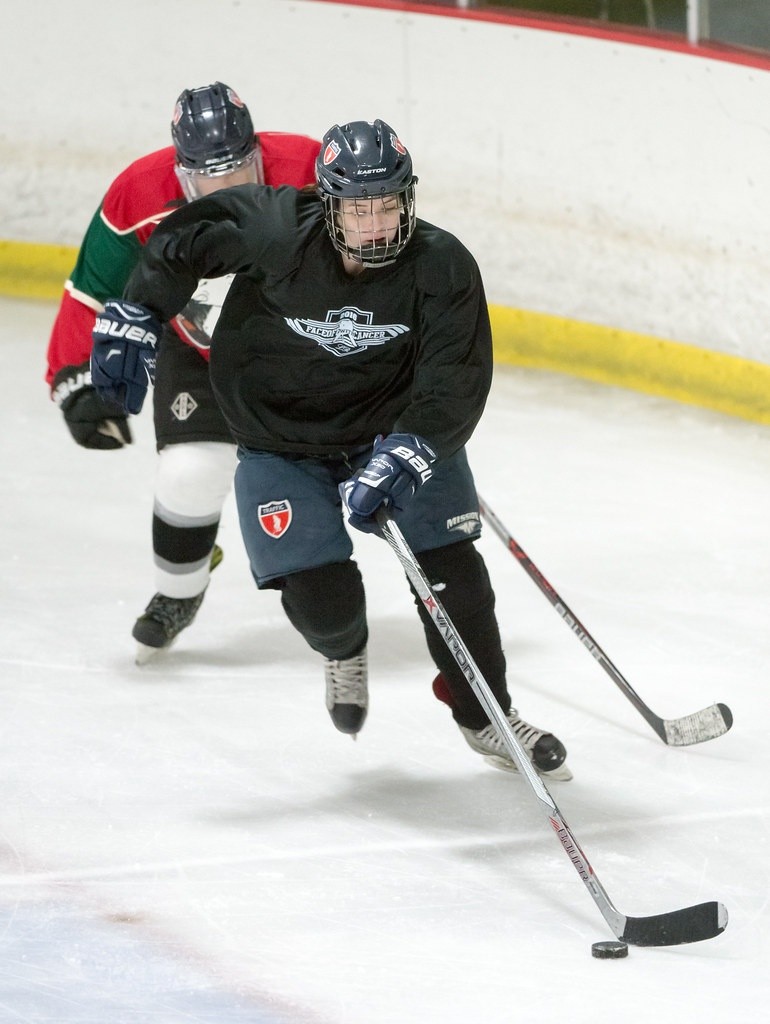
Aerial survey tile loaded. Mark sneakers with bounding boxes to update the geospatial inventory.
[133,545,224,665]
[433,673,574,781]
[324,646,368,739]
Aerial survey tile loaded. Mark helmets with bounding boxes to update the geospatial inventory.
[314,119,417,269]
[171,80,257,176]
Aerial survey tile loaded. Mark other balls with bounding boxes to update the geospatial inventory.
[592,941,629,959]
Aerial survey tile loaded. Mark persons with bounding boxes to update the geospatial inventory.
[48,82,325,668]
[90,118,572,782]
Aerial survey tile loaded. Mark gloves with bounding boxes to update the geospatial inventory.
[90,299,164,414]
[51,360,132,448]
[343,432,438,534]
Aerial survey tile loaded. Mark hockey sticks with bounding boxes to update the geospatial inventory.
[352,467,731,947]
[476,496,734,748]
